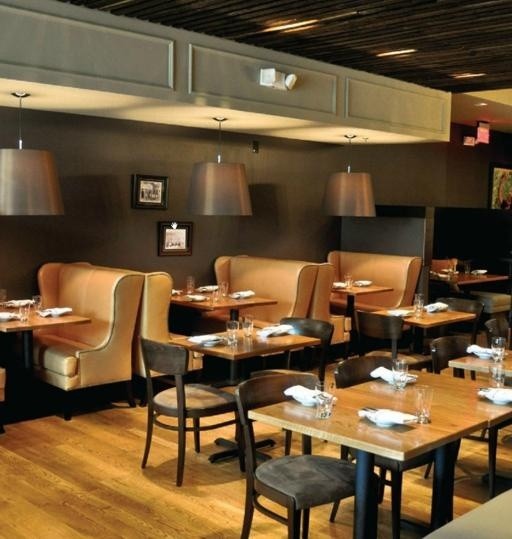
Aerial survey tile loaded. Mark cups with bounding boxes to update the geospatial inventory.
[241,314,254,336]
[488,364,506,388]
[392,358,408,390]
[414,385,436,424]
[226,320,239,345]
[492,335,506,360]
[313,381,335,419]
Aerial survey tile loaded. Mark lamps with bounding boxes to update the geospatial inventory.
[324,133,375,217]
[191,116,252,216]
[0,92,64,215]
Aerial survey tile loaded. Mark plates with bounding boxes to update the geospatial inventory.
[172,275,255,303]
[370,418,395,427]
[473,352,491,359]
[292,395,314,406]
[0,287,74,324]
[199,341,220,347]
[388,292,448,318]
[436,259,488,277]
[488,396,512,405]
[332,273,373,288]
[384,378,408,385]
[270,331,288,336]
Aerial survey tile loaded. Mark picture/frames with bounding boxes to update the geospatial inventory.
[159,220,194,256]
[130,172,169,211]
